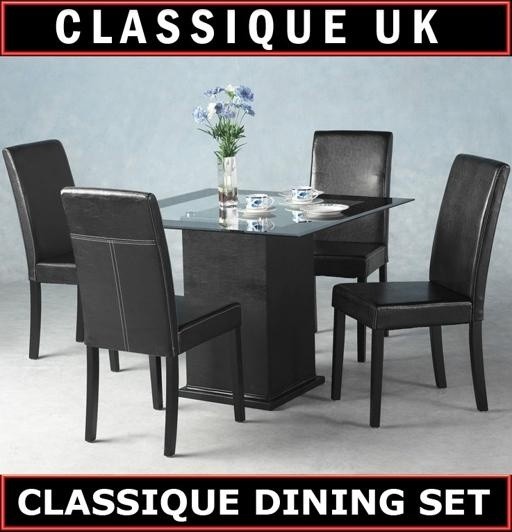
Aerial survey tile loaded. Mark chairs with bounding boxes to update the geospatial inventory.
[304,130,393,365]
[329,152,512,429]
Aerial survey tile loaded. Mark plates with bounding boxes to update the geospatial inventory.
[239,206,278,215]
[277,191,326,197]
[298,202,350,218]
[280,199,327,205]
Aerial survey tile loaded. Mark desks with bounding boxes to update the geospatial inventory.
[138,183,416,413]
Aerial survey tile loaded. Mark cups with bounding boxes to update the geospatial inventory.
[246,219,275,235]
[242,194,275,209]
[286,187,318,202]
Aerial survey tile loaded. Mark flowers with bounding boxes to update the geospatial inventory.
[194,80,257,153]
